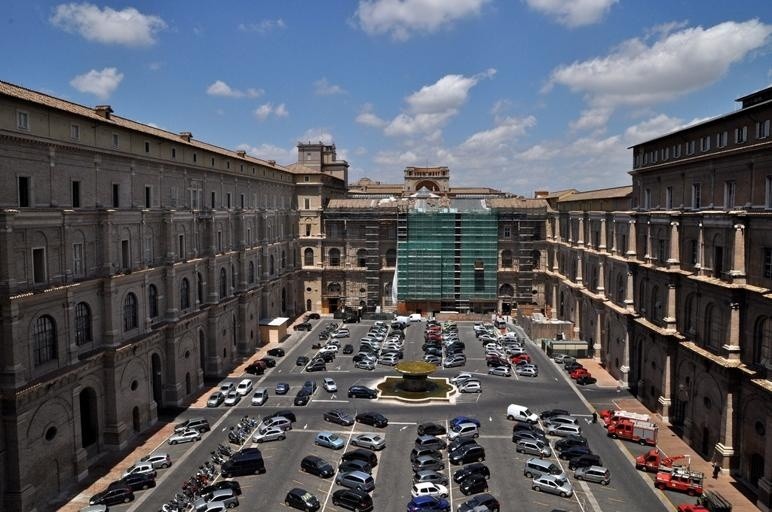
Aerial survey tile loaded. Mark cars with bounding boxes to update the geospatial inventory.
[207,313,422,412]
[420,314,467,368]
[474,312,539,376]
[284,408,389,512]
[554,352,596,386]
[449,373,482,394]
[406,416,502,512]
[87,410,297,512]
[504,403,730,512]
